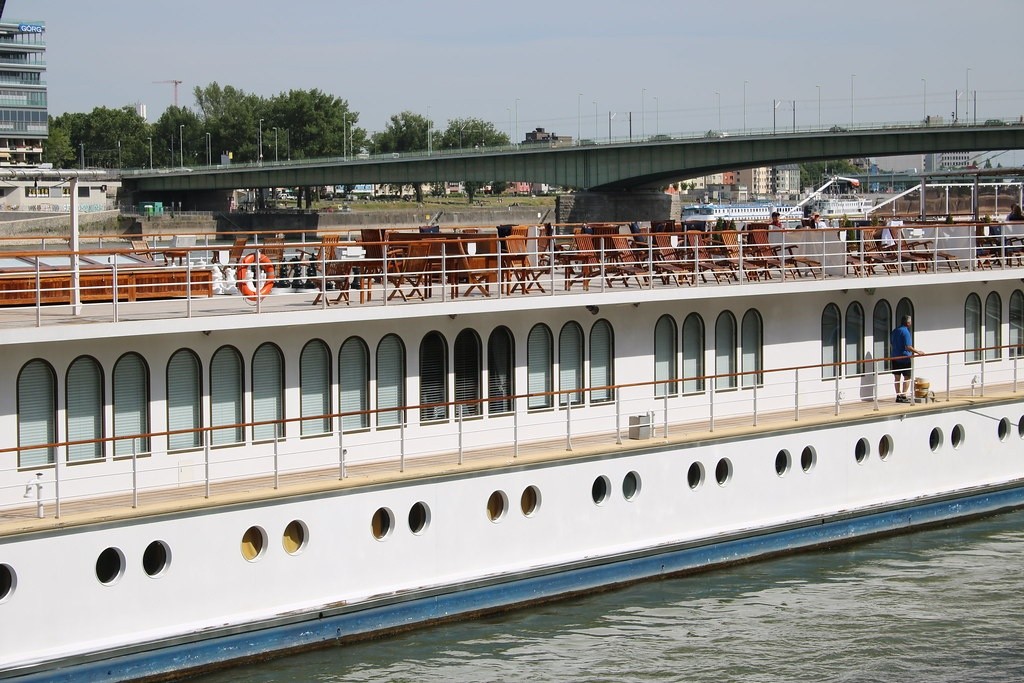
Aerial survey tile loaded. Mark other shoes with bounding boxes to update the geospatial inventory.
[896,397,911,403]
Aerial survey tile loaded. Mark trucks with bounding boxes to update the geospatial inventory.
[904,168,917,173]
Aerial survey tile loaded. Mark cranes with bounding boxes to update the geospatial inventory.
[152,80,182,106]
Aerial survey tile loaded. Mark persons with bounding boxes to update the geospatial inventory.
[1008,206,1024,222]
[810,212,827,229]
[890,314,925,403]
[770,212,784,231]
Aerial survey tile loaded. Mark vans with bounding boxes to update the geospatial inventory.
[38,163,53,169]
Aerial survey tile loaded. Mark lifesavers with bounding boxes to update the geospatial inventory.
[237,253,274,301]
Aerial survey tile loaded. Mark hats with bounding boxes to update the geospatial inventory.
[772,212,780,217]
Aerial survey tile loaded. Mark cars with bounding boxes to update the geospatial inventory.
[580,139,599,145]
[649,134,674,140]
[829,127,846,132]
[984,119,1007,125]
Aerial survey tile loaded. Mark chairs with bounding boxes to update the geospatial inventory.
[846,226,958,277]
[976,224,1024,271]
[211,237,246,265]
[259,238,286,281]
[294,219,822,306]
[132,239,166,267]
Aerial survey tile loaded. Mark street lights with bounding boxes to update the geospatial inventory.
[921,78,926,127]
[206,133,211,165]
[272,127,277,161]
[348,121,353,161]
[641,80,821,141]
[343,112,348,161]
[180,125,184,167]
[260,119,264,166]
[966,68,971,127]
[79,144,85,170]
[147,138,152,169]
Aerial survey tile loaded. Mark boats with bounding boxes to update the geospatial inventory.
[0,182,1024,683]
[680,174,873,230]
